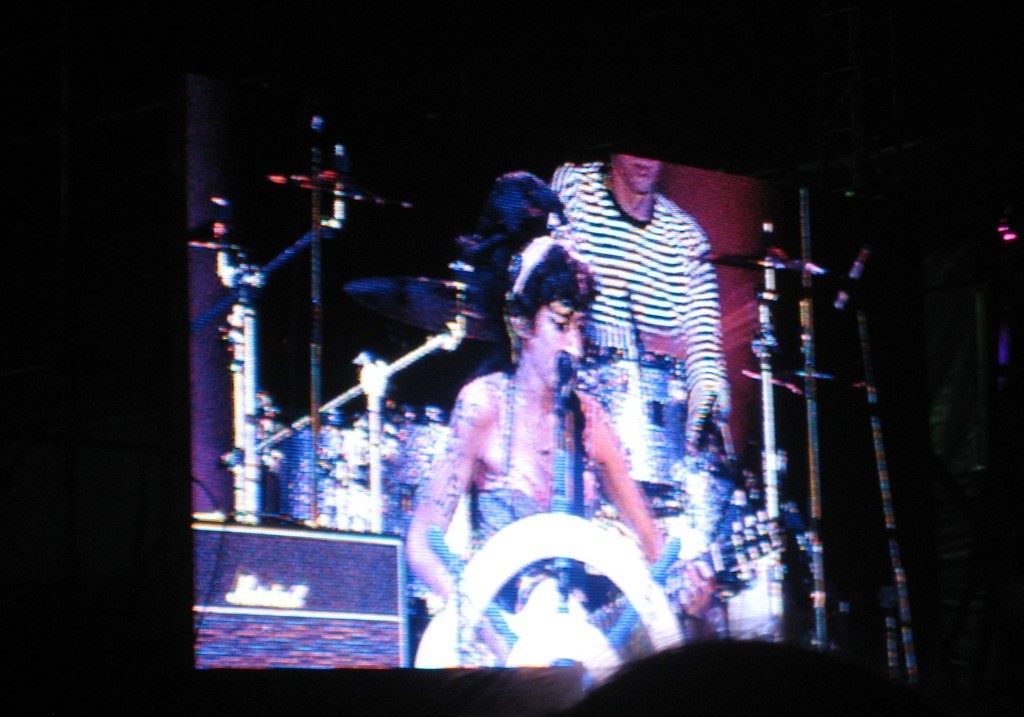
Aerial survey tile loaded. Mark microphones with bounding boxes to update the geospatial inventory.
[835,247,871,309]
[554,351,574,387]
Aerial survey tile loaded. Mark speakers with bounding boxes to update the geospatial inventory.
[191,522,410,672]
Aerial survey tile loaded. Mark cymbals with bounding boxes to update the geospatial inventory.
[706,250,825,277]
[265,171,415,210]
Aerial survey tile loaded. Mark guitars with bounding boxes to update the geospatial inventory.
[413,500,814,669]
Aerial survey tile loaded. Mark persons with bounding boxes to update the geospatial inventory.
[405,171,717,666]
[559,639,887,717]
[547,152,734,642]
